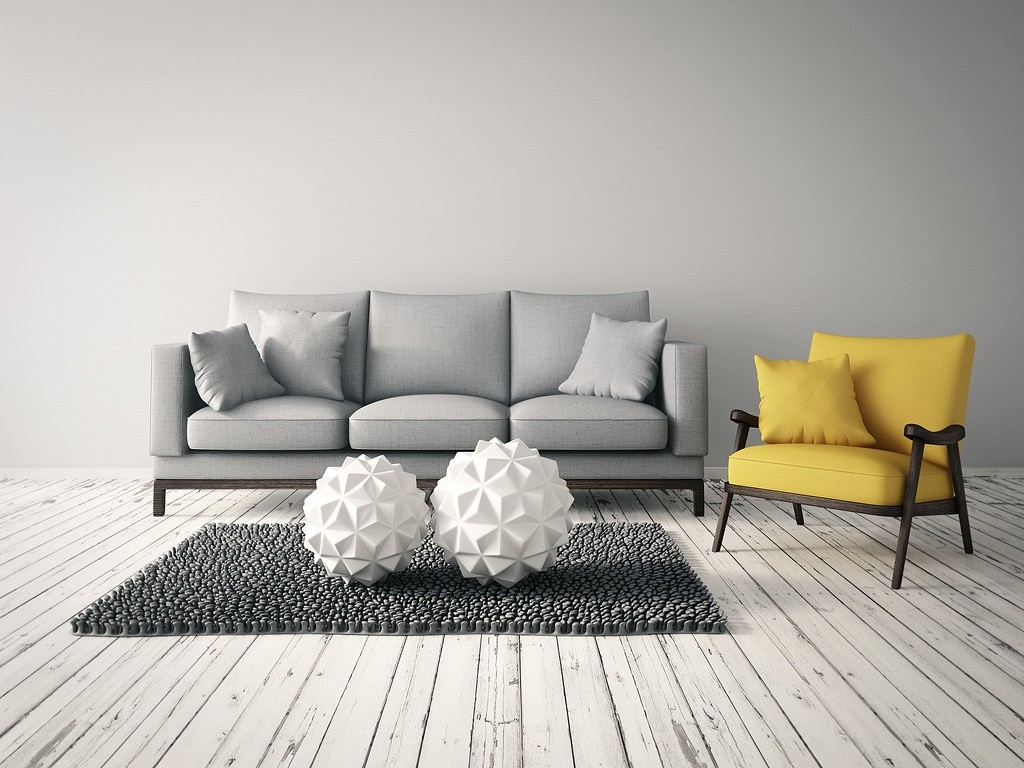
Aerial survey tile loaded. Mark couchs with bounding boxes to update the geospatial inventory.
[147,289,707,517]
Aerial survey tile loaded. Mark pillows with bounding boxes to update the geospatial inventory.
[559,313,667,402]
[188,323,286,412]
[754,353,876,448]
[256,309,350,402]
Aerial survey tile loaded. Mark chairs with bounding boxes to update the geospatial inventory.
[712,330,975,589]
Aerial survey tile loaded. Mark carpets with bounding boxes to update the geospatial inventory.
[69,523,725,635]
